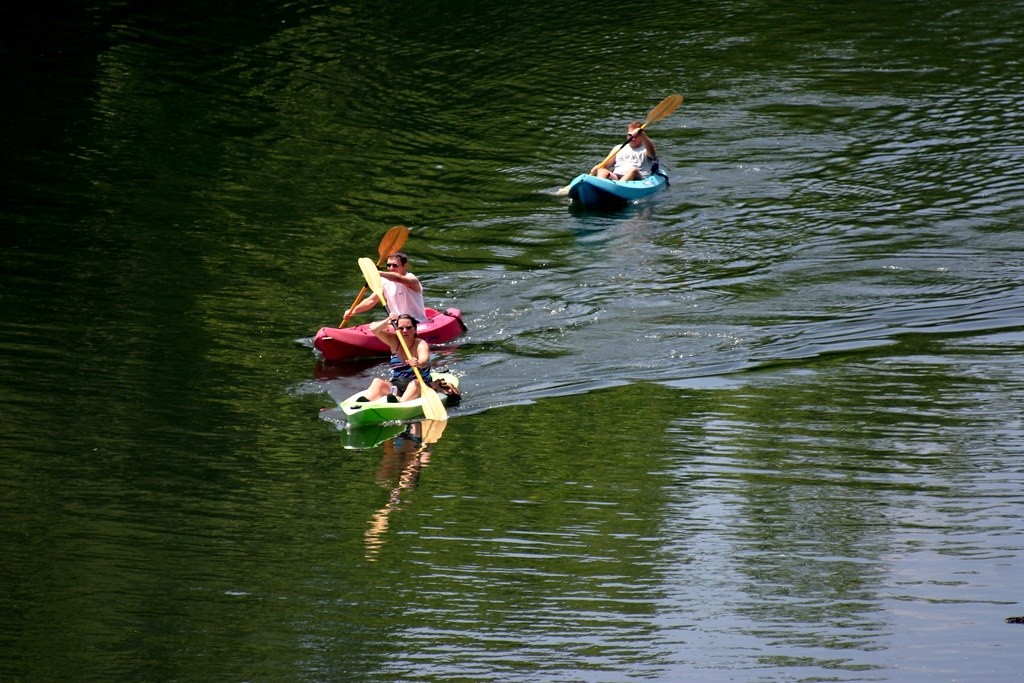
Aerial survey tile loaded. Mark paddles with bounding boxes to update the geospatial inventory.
[552,93,684,198]
[339,225,448,421]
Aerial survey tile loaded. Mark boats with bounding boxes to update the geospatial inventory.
[339,367,459,426]
[567,166,667,208]
[313,300,464,360]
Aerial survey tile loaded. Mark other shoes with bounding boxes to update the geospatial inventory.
[349,396,370,409]
[386,394,398,403]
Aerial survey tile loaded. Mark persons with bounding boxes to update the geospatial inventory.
[591,121,656,181]
[344,252,427,324]
[351,313,431,409]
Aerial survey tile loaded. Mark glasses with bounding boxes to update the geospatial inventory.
[627,132,633,136]
[386,263,400,267]
[398,325,413,330]
